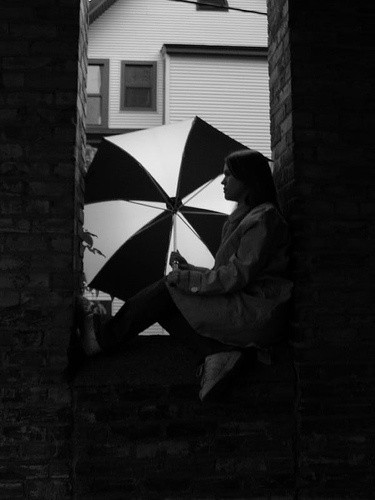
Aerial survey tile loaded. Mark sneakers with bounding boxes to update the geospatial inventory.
[78,295,101,357]
[197,349,241,400]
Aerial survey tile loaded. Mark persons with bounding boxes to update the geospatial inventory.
[83,149,294,401]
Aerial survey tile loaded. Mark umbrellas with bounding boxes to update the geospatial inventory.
[84,116,274,302]
